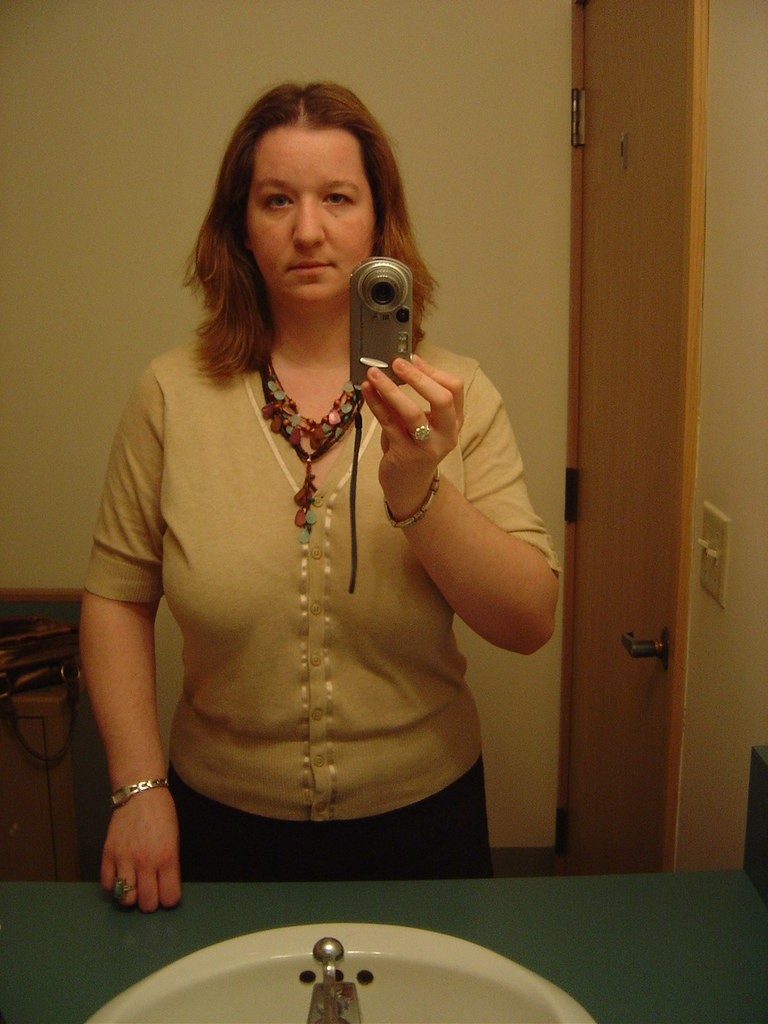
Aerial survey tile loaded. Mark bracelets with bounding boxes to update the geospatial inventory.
[110,778,169,806]
[384,467,439,528]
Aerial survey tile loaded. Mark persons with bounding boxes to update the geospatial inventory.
[78,82,559,912]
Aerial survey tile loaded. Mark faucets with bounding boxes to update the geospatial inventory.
[306,938,361,1023]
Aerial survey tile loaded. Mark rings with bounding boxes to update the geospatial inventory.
[113,878,136,900]
[410,422,431,441]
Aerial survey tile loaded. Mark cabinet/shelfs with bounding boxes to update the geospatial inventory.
[0,647,82,883]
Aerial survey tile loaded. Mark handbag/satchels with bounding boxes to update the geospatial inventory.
[0,613,82,760]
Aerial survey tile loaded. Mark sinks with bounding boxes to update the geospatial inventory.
[85,921,594,1023]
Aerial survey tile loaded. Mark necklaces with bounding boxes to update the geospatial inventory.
[259,353,364,543]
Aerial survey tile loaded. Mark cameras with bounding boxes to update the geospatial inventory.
[349,256,413,386]
